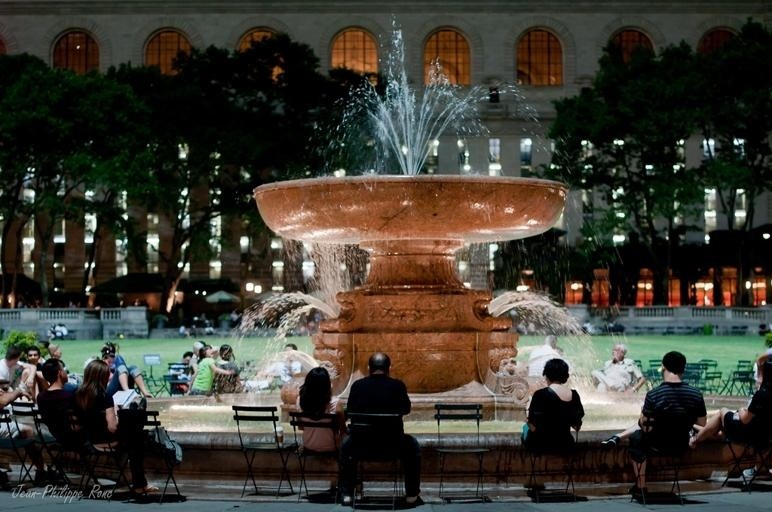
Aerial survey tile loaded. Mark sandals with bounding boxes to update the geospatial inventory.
[134,484,159,494]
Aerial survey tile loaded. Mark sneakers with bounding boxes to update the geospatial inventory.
[628,484,649,499]
[601,436,620,450]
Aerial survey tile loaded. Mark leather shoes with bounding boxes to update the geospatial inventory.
[404,494,424,508]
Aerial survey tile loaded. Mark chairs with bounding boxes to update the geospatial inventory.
[0,388,33,493]
[287,411,343,503]
[11,399,73,494]
[434,403,486,501]
[71,404,134,502]
[628,357,759,399]
[631,408,700,506]
[342,408,406,511]
[232,405,295,499]
[523,415,583,504]
[722,412,772,493]
[114,407,183,504]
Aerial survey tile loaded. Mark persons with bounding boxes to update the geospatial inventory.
[183,351,194,364]
[74,359,159,494]
[295,366,344,493]
[592,345,645,392]
[603,351,707,497]
[583,317,626,336]
[519,335,564,377]
[21,346,50,401]
[35,358,79,450]
[521,359,585,489]
[339,351,423,508]
[190,342,204,366]
[101,353,152,398]
[212,344,243,393]
[0,388,57,484]
[189,345,236,396]
[261,344,301,374]
[691,355,772,479]
[48,343,62,360]
[180,311,242,338]
[1,343,38,400]
